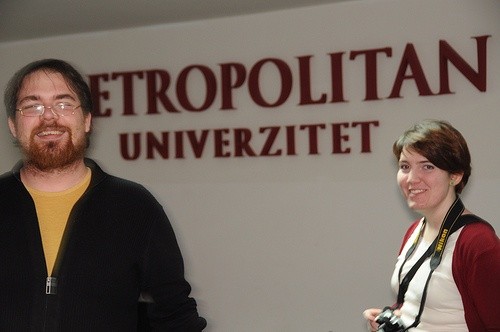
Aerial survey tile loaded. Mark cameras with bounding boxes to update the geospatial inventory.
[374,311,405,332]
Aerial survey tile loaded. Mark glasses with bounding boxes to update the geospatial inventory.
[14,101,83,116]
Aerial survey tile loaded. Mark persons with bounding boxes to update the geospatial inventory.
[1,57,208,332]
[357,119,500,332]
